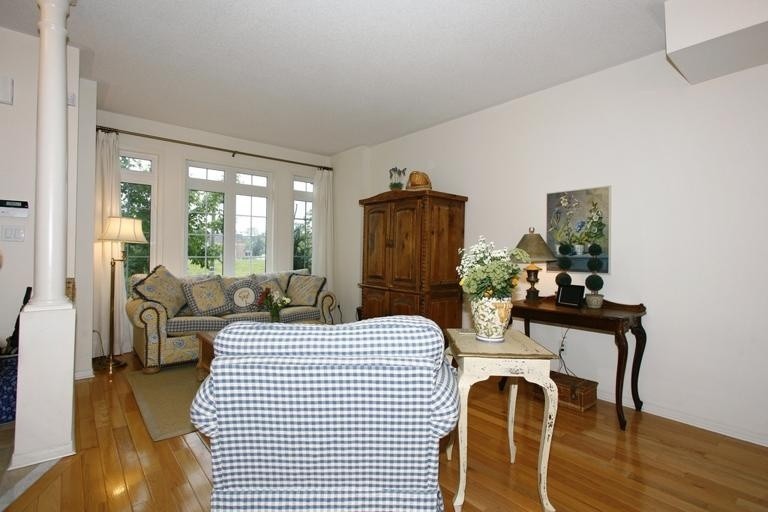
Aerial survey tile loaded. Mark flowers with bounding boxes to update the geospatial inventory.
[547,192,606,244]
[455,234,532,300]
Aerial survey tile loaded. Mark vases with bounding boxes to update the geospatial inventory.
[556,245,560,254]
[575,244,584,255]
[471,299,510,343]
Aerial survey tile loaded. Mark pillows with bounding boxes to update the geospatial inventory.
[182,274,233,316]
[285,272,326,307]
[226,277,288,314]
[132,264,187,319]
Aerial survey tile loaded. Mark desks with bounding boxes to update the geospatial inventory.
[512,295,647,431]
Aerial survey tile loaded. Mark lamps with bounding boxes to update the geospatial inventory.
[510,227,558,301]
[97,217,149,369]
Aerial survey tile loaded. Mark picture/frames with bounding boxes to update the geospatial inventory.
[556,285,584,307]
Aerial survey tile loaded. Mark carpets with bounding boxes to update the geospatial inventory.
[127,364,198,442]
[0,419,62,511]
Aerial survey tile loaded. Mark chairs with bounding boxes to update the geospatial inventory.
[189,314,460,511]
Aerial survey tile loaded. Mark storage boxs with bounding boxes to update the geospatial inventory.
[532,370,599,413]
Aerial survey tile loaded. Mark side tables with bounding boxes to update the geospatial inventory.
[444,328,559,496]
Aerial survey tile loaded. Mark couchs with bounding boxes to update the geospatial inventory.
[125,268,337,374]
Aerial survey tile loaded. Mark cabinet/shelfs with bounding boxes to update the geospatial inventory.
[357,190,467,349]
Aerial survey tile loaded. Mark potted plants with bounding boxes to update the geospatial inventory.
[250,288,292,322]
[585,244,604,308]
[389,167,407,191]
